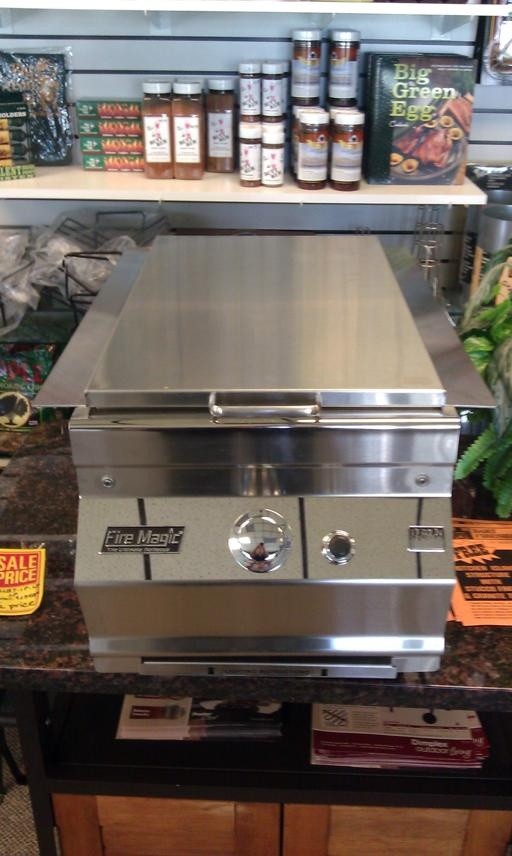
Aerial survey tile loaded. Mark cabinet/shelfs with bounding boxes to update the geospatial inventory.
[1,0,511,205]
[44,693,512,855]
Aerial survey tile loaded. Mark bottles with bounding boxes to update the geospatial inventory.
[289,29,365,192]
[205,78,237,173]
[143,78,205,180]
[238,60,290,189]
